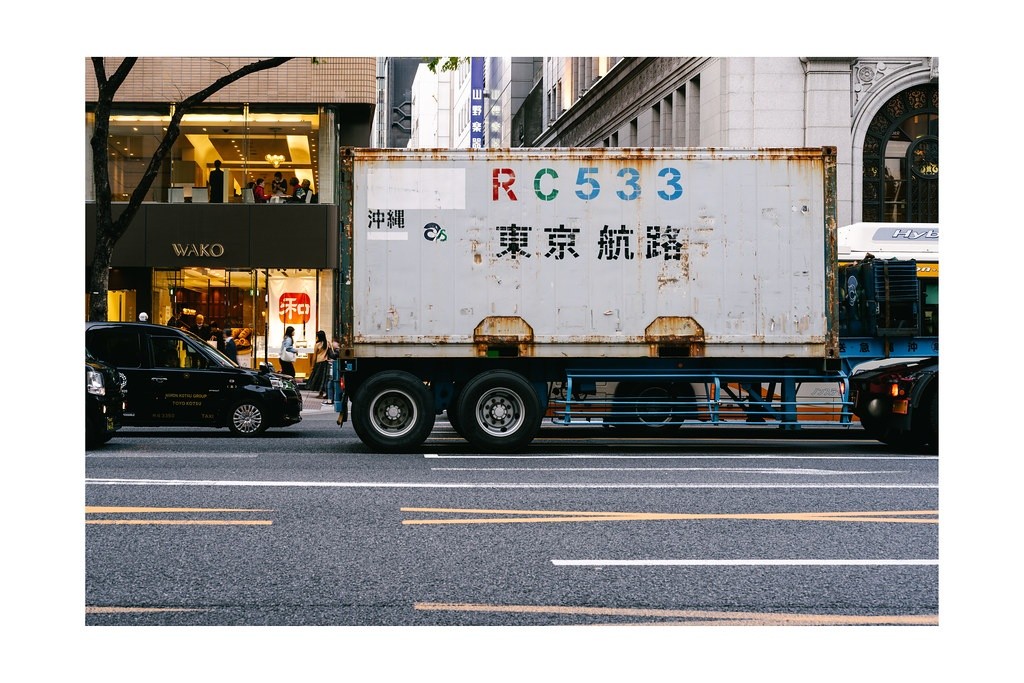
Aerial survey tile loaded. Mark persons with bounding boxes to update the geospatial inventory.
[237,172,313,203]
[167,308,182,327]
[305,330,339,407]
[190,314,239,369]
[209,160,224,203]
[279,326,297,378]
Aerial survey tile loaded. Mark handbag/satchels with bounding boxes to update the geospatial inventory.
[327,341,339,360]
[280,347,297,362]
[207,338,217,350]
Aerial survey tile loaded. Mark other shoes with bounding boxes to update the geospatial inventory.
[316,395,323,399]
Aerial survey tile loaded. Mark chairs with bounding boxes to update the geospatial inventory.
[192,187,209,202]
[244,188,255,204]
[305,190,313,203]
[241,187,246,202]
[168,187,184,203]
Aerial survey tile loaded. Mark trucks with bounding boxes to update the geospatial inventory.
[339,144,938,452]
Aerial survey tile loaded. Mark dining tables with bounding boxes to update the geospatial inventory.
[264,195,293,204]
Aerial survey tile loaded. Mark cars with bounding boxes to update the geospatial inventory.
[85,321,303,436]
[86,350,132,451]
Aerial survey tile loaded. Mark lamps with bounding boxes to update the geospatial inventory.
[265,128,285,169]
